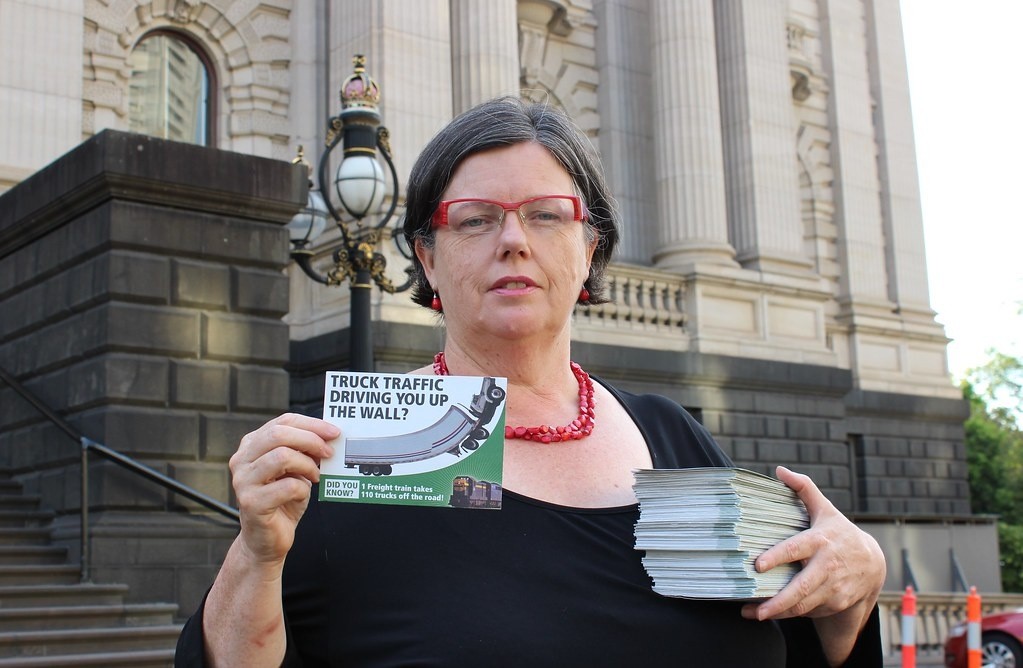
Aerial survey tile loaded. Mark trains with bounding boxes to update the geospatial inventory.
[448,474,502,510]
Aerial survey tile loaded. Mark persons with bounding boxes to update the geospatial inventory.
[170,94,890,666]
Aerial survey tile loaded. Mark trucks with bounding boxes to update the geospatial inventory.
[343,376,506,477]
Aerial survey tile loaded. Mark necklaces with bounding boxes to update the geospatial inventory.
[428,347,595,446]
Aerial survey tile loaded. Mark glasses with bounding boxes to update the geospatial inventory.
[429,194,592,237]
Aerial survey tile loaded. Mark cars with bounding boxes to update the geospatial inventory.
[944,608,1023,668]
